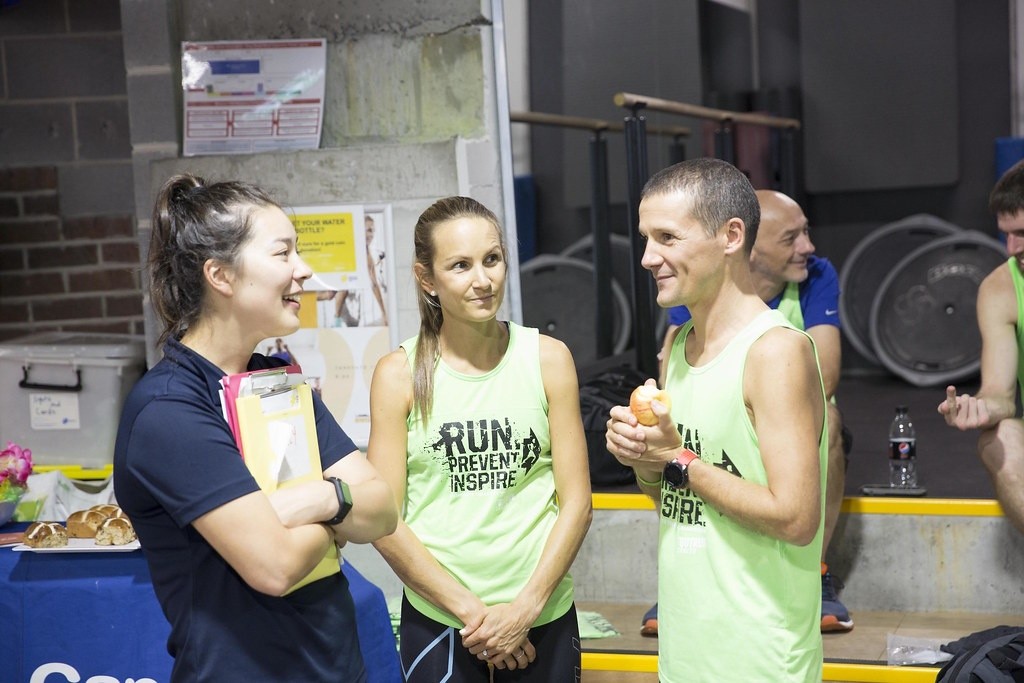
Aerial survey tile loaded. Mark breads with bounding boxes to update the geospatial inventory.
[23,505,139,548]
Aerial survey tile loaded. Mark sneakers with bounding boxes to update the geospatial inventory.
[821,562,853,630]
[640,602,658,634]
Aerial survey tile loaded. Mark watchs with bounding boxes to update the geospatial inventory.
[321,477,353,525]
[662,449,701,491]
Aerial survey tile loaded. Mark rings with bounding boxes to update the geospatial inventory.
[482,650,490,658]
[515,650,525,660]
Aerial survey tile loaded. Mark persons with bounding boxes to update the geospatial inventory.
[366,190,593,683]
[113,176,397,683]
[638,189,854,636]
[264,337,298,368]
[318,214,390,330]
[935,160,1024,534]
[603,156,829,683]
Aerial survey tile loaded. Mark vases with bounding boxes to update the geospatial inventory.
[0,496,23,527]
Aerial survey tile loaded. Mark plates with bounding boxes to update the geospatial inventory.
[0,538,141,553]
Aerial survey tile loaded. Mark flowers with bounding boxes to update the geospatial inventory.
[0,440,34,503]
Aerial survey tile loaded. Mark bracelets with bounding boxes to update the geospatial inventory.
[635,471,663,488]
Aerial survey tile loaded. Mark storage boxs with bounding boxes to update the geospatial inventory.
[0,332,145,469]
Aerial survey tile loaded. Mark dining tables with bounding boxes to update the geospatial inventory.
[0,521,405,683]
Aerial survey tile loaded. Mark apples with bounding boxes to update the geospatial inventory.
[630,386,672,425]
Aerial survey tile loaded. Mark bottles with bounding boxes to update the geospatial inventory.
[889,406,917,490]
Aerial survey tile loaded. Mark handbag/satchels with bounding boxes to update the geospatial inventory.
[578,365,654,486]
